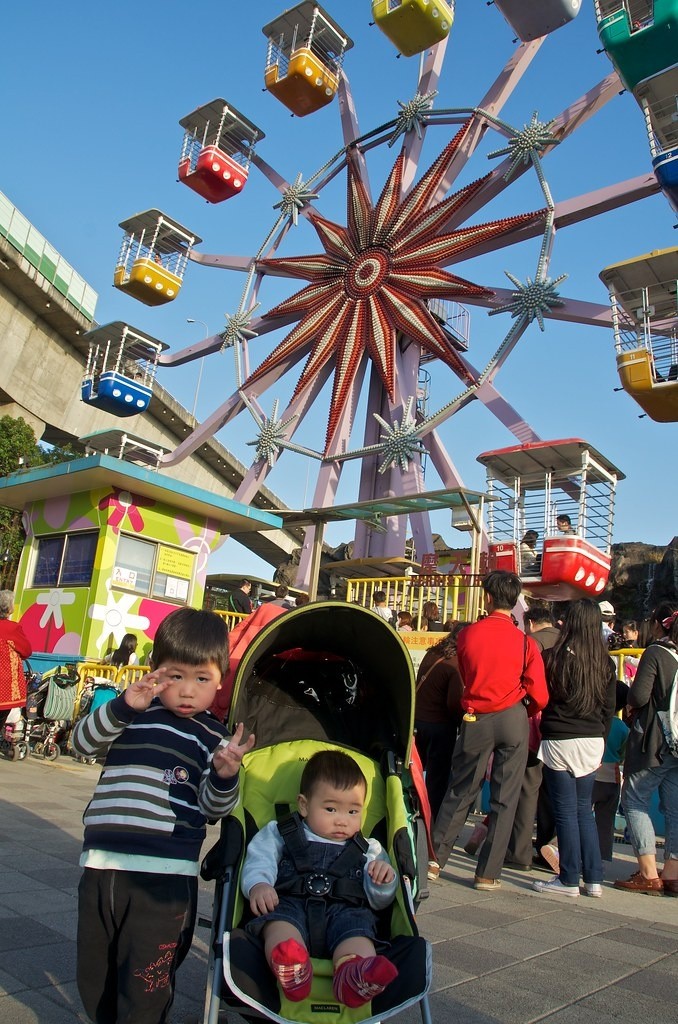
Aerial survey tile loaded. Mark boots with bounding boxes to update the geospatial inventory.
[464,822,488,855]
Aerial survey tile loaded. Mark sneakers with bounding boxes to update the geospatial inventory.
[658,871,678,897]
[533,876,580,898]
[614,871,665,897]
[585,883,602,898]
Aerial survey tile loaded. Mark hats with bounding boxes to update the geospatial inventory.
[598,601,616,615]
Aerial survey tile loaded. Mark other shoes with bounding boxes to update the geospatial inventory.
[474,875,502,890]
[541,845,560,874]
[503,860,532,871]
[427,863,440,880]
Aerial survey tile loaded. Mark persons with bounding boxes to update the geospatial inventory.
[70,607,256,1024]
[520,530,540,573]
[269,584,310,610]
[128,373,142,380]
[413,570,678,898]
[369,590,458,631]
[228,577,252,631]
[556,514,576,535]
[154,253,162,267]
[240,750,402,1008]
[98,634,140,690]
[0,589,33,732]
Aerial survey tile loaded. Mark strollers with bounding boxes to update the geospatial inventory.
[1,658,81,761]
[202,602,435,1021]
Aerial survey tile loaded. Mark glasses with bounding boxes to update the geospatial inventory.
[513,621,518,626]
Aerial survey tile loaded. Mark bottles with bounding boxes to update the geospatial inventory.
[462,707,476,722]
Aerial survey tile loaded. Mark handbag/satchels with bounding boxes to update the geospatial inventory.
[43,677,75,721]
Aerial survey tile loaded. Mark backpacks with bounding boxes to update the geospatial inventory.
[646,644,678,758]
